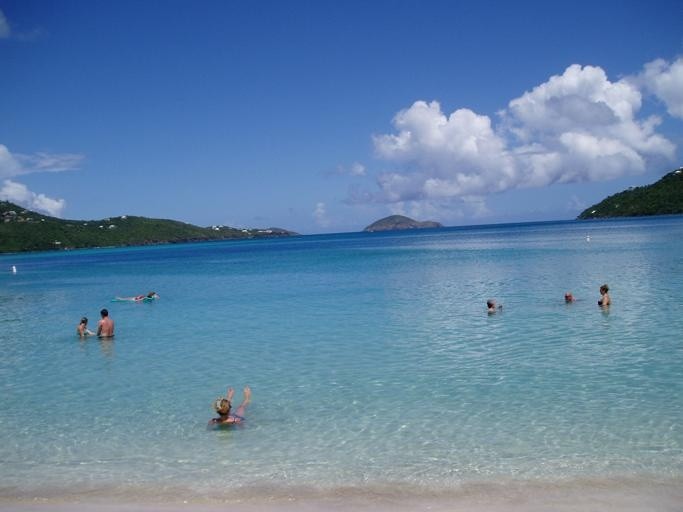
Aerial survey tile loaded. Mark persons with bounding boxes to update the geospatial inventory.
[485,298,503,314]
[76,316,95,337]
[96,308,114,338]
[206,384,252,428]
[114,292,161,304]
[564,292,577,302]
[597,283,610,306]
